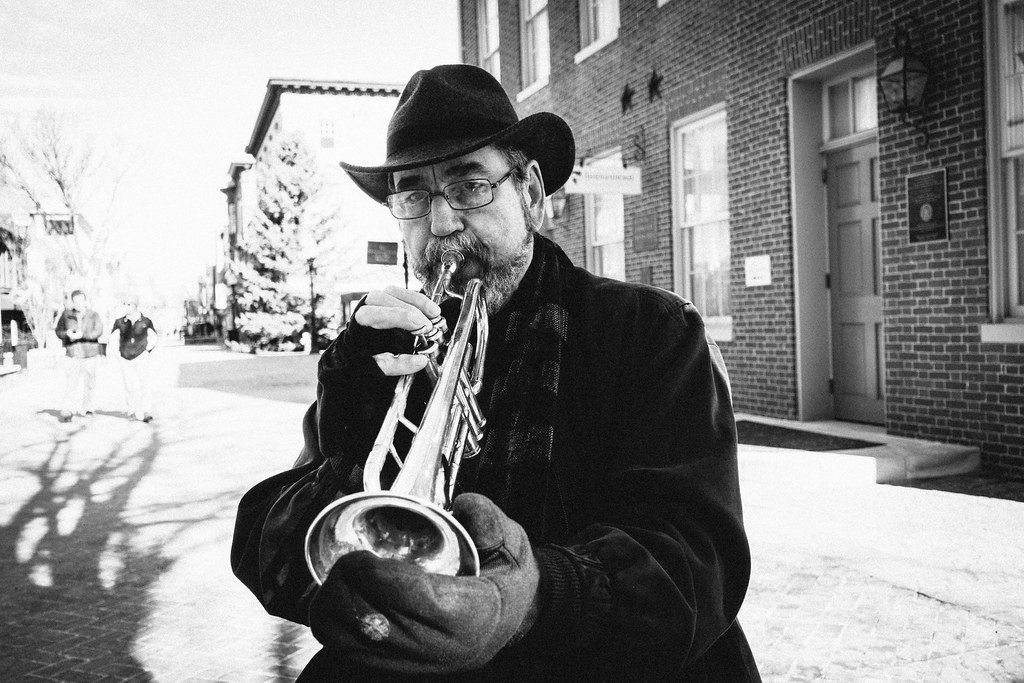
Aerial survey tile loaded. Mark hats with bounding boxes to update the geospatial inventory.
[339,64,575,206]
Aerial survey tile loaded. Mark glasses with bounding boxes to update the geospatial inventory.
[382,167,517,220]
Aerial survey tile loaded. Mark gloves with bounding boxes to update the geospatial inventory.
[308,492,540,675]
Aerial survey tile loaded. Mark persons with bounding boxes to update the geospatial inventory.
[110,297,159,424]
[54,290,103,423]
[229,63,762,683]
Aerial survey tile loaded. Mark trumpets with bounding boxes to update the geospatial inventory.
[302,250,491,605]
